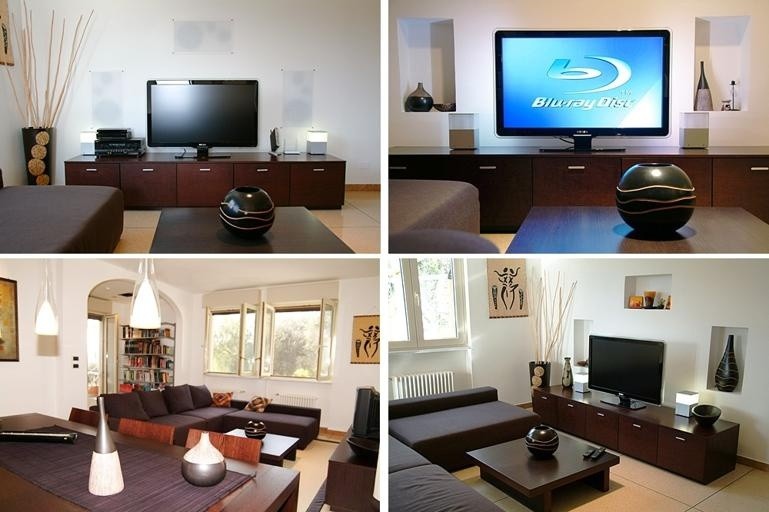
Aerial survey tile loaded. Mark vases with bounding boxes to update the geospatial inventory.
[528,360,551,402]
[22,125,56,185]
[88,396,125,495]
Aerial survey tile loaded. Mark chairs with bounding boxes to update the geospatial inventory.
[68,406,109,428]
[116,417,175,447]
[184,428,263,462]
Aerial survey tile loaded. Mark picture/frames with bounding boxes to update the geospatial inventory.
[0,277,20,363]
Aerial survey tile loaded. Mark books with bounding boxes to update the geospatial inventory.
[121,325,174,391]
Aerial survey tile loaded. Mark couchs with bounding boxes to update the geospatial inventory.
[388,385,546,512]
[90,383,320,452]
[389,177,479,235]
[0,167,126,253]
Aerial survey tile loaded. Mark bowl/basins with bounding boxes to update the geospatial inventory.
[691,404,722,427]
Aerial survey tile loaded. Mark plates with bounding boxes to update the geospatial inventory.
[431,101,456,112]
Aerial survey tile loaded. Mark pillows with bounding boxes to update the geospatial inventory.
[210,391,234,408]
[244,396,272,413]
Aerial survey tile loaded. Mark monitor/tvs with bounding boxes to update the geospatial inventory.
[588,335,664,410]
[492,28,674,153]
[352,386,380,439]
[147,80,259,159]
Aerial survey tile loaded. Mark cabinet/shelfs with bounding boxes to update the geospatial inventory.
[121,321,177,393]
[532,154,621,206]
[389,154,452,178]
[451,155,532,233]
[326,425,380,512]
[176,162,234,209]
[622,154,714,207]
[65,162,120,189]
[234,162,290,206]
[290,162,346,209]
[714,155,769,225]
[658,425,740,485]
[586,405,618,452]
[121,161,176,208]
[559,396,586,440]
[618,414,658,467]
[533,388,559,433]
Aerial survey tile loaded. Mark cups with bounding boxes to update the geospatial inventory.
[644,290,656,307]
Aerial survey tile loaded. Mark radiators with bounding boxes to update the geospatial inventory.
[276,391,318,408]
[388,370,455,401]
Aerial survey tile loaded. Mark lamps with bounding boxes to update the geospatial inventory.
[80,131,95,158]
[33,258,59,338]
[306,129,329,155]
[679,111,709,149]
[572,371,590,393]
[674,391,699,417]
[448,112,480,150]
[130,257,162,331]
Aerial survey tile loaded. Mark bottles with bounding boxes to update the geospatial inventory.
[529,361,552,388]
[245,420,267,440]
[615,161,698,239]
[525,424,560,459]
[217,185,276,238]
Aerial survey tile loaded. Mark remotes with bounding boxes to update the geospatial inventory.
[175,152,184,158]
[583,449,595,457]
[590,447,606,459]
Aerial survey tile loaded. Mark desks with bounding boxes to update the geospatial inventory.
[147,206,356,254]
[0,411,301,512]
